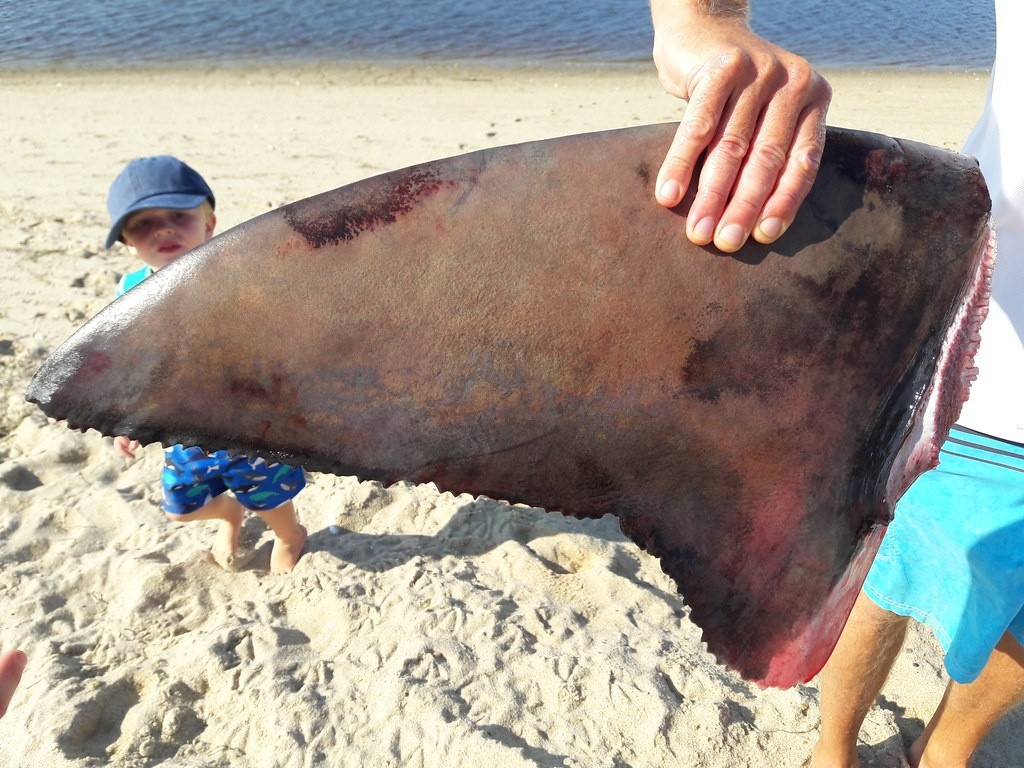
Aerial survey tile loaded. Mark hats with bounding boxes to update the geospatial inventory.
[105,155,216,249]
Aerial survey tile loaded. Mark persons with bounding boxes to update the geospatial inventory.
[105,156,308,576]
[651,0,1023,768]
